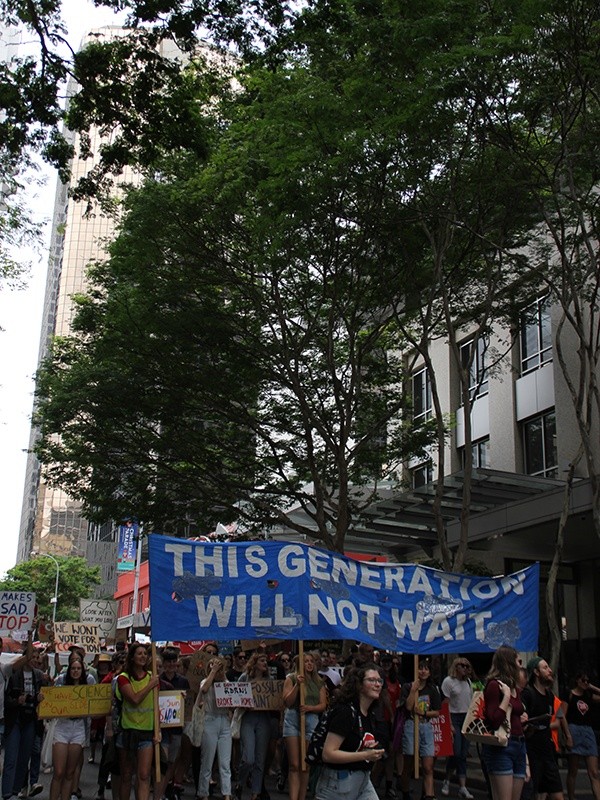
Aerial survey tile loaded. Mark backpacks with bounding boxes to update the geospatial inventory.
[305,702,375,765]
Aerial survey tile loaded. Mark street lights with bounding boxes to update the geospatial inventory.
[30,550,59,621]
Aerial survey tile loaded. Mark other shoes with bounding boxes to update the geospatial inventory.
[378,779,473,800]
[18,757,288,800]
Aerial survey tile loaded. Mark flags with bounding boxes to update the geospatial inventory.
[115,514,139,572]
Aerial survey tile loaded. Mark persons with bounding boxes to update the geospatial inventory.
[0,637,442,800]
[439,657,474,799]
[312,661,385,800]
[476,645,600,800]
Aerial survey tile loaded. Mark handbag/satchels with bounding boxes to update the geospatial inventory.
[529,727,566,761]
[186,684,209,747]
[460,691,512,747]
[41,721,53,768]
[229,675,249,739]
[389,712,406,753]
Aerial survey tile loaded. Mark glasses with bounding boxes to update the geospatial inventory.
[580,677,590,684]
[519,660,522,665]
[207,652,216,655]
[363,678,384,685]
[457,663,469,669]
[239,656,248,660]
[281,659,290,663]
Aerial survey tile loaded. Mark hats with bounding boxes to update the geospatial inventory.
[68,646,85,656]
[98,654,112,662]
[382,654,391,661]
[22,641,44,655]
[527,657,542,677]
